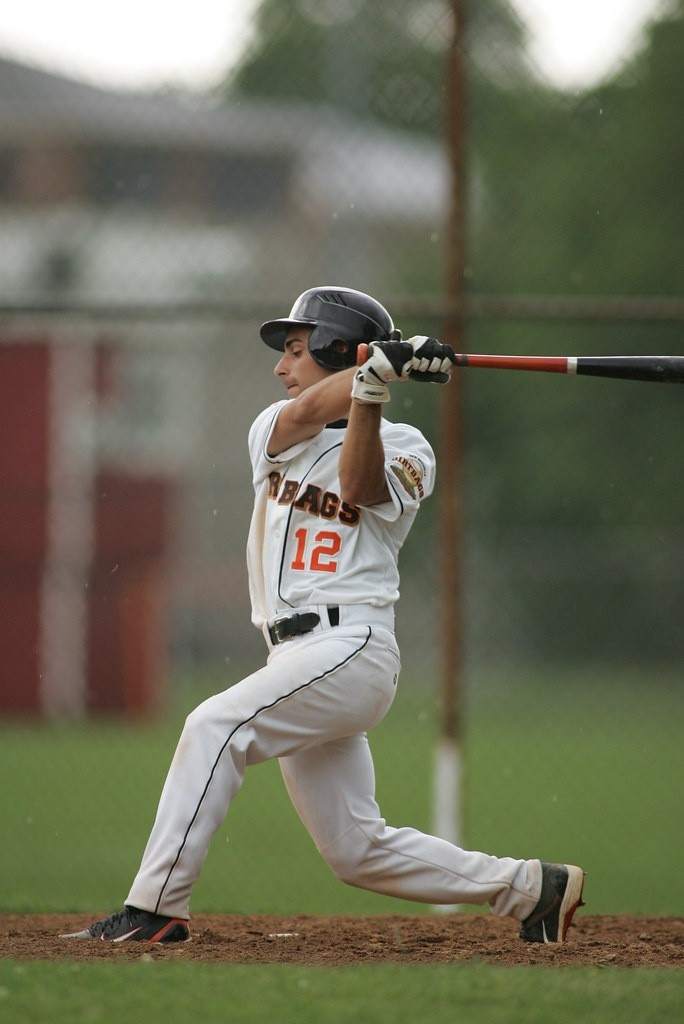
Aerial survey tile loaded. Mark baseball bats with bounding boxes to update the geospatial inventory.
[353,342,684,387]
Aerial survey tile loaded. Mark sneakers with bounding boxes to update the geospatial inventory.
[520,859,586,947]
[59,904,192,944]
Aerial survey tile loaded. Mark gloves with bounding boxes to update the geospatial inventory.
[391,328,459,385]
[351,339,413,404]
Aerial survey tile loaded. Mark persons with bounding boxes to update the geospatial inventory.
[59,286,588,945]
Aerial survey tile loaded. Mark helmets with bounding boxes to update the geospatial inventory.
[260,286,395,369]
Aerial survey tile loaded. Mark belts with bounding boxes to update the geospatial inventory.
[269,606,339,645]
